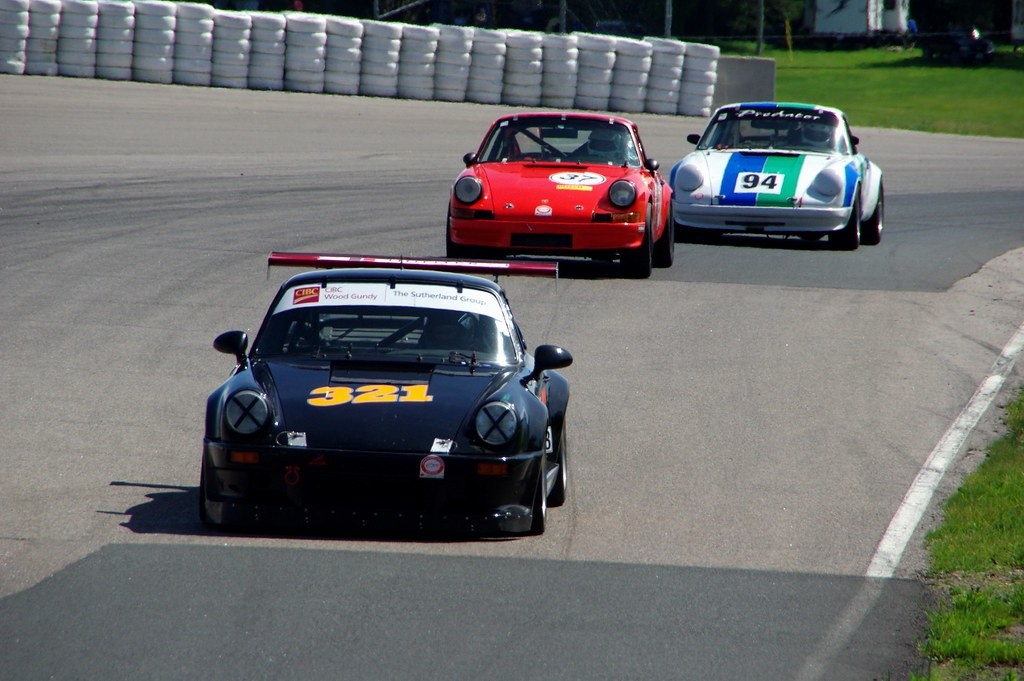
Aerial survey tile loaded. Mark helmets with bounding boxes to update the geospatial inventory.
[802,123,832,147]
[587,129,620,160]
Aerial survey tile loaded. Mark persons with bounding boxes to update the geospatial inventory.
[1009,17,1024,57]
[900,15,920,53]
[418,311,478,358]
[799,123,832,149]
[584,130,624,165]
[961,29,996,69]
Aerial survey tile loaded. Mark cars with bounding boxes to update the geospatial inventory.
[198,248,574,534]
[670,102,884,251]
[446,112,676,278]
